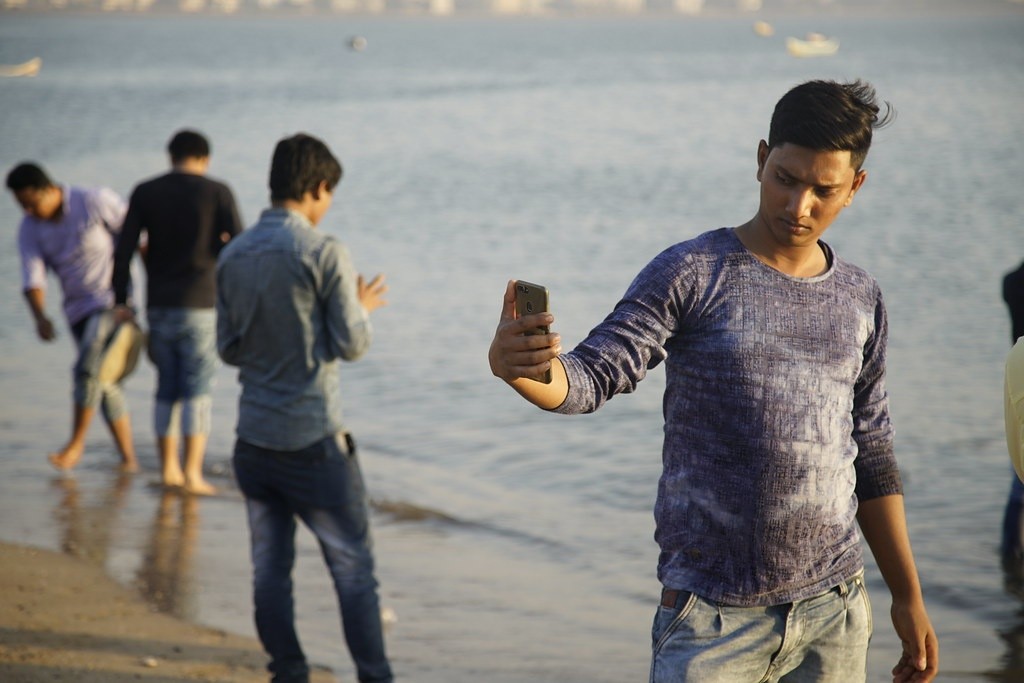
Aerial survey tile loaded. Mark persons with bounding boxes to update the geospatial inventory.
[487,77,1024,683]
[44,474,198,620]
[214,132,400,683]
[4,160,149,473]
[111,129,250,497]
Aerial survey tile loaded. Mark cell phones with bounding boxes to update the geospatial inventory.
[515,280,553,385]
